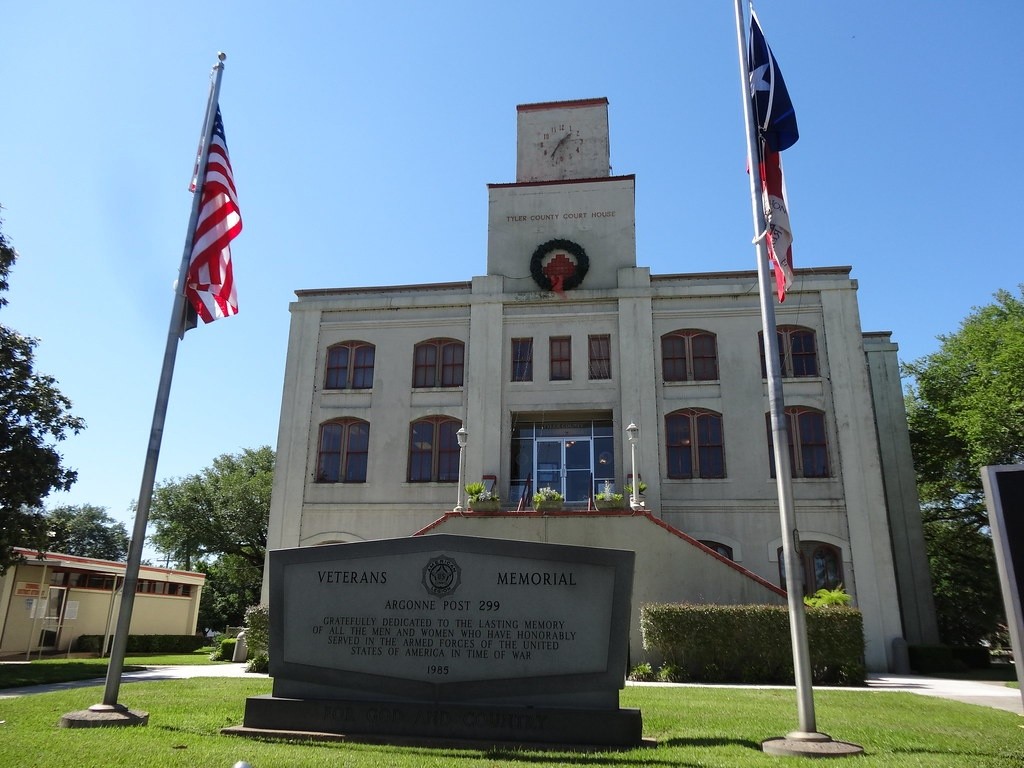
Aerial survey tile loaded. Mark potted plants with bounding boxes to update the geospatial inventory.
[465,481,502,511]
[625,480,647,497]
[594,493,624,511]
[531,487,563,512]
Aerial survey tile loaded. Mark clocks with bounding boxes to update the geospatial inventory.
[538,122,585,166]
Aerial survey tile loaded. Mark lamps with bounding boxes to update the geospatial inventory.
[626,420,639,443]
[456,424,468,447]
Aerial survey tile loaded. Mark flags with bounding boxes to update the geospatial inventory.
[747,8,800,303]
[179,84,244,341]
